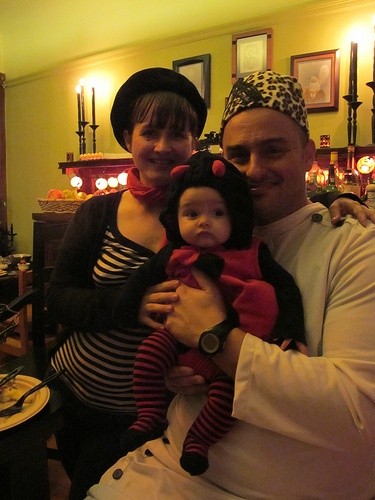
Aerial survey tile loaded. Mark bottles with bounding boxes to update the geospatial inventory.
[305,145,360,198]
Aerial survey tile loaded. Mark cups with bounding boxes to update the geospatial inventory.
[319,135,330,148]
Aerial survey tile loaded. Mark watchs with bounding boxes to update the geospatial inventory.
[198,318,237,356]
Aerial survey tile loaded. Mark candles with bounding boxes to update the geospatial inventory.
[77,94,81,131]
[349,41,357,94]
[92,88,95,125]
[81,86,85,122]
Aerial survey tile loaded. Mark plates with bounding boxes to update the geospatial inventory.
[0,374,51,431]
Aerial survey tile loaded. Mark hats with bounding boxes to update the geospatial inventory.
[219,70,309,149]
[109,66,208,153]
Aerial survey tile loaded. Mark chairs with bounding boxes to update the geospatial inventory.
[0,268,63,367]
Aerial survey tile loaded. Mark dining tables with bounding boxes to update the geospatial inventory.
[0,368,60,500]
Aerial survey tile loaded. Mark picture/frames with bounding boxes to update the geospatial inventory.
[231,27,272,84]
[173,53,211,108]
[290,49,340,114]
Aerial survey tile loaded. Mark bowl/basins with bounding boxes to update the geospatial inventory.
[13,254,32,263]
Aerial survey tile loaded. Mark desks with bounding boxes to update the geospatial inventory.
[58,145,375,195]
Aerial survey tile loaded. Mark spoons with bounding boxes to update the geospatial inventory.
[0,369,66,415]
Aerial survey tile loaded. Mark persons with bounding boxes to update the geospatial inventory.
[105,151,311,477]
[83,68,375,500]
[41,66,375,500]
